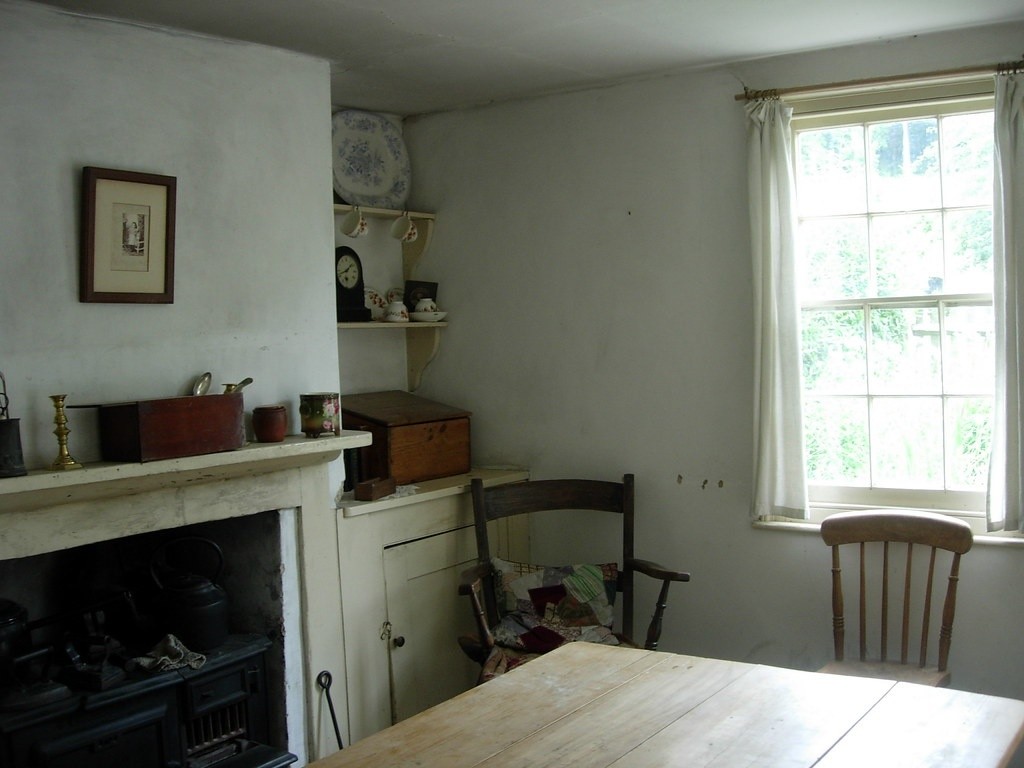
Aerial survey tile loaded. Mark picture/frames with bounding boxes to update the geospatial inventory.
[79,166,178,306]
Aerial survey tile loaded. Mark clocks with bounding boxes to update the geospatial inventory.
[335,246,365,322]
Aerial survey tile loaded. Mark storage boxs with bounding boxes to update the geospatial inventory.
[340,391,474,485]
[65,393,246,462]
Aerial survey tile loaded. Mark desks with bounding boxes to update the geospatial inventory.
[301,640,1024,768]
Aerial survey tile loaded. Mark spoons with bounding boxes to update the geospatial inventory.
[193,372,212,396]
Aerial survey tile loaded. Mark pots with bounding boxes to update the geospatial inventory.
[0,597,49,688]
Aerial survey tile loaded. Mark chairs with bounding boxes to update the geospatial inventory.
[457,473,691,666]
[814,510,974,689]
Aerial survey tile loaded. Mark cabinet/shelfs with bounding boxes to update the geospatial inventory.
[333,203,448,392]
[337,466,531,748]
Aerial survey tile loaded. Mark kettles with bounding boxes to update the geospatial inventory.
[132,535,228,651]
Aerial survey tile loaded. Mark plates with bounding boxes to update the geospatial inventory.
[333,110,411,209]
[365,287,387,309]
[410,311,448,322]
[388,288,404,303]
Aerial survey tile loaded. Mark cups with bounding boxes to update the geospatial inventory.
[341,206,368,238]
[391,212,417,242]
[416,299,439,312]
[385,302,409,321]
